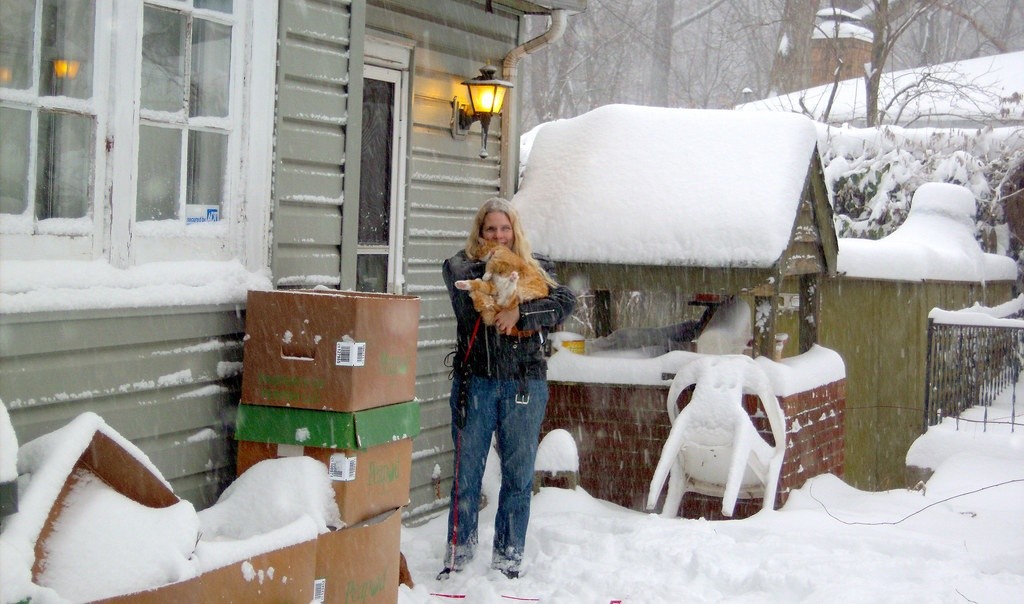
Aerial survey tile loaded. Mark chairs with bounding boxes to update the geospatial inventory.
[645,354,786,519]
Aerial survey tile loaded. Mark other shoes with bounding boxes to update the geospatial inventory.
[436,567,461,581]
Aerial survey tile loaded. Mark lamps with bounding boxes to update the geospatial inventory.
[45,37,87,81]
[452,58,514,159]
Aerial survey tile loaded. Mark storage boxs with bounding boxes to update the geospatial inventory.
[86,539,314,604]
[241,288,421,412]
[316,506,402,604]
[0,411,202,604]
[234,402,421,527]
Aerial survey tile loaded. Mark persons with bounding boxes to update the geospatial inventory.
[429,199,576,584]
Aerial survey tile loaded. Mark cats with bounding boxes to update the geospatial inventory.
[456,235,549,337]
